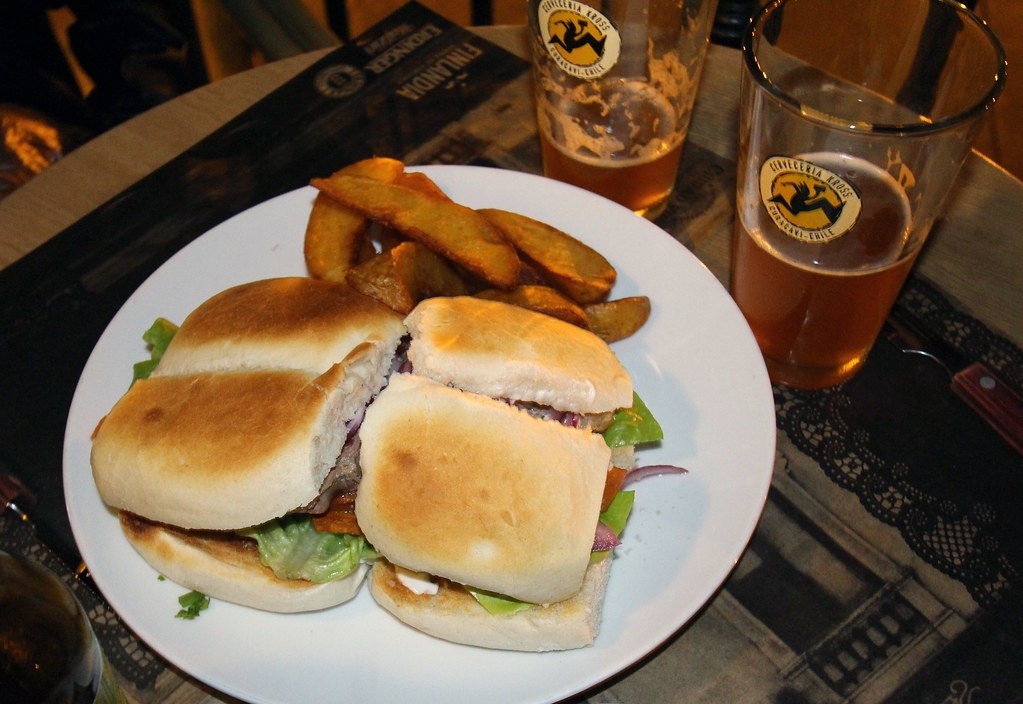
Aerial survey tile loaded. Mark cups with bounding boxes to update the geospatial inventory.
[523,0,714,221]
[729,3,1007,387]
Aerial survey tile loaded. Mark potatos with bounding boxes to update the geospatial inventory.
[301,156,650,345]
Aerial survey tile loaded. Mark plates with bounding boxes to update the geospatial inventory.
[60,168,773,704]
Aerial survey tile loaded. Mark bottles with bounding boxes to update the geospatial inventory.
[3,546,105,703]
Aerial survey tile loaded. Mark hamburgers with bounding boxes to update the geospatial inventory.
[87,277,686,653]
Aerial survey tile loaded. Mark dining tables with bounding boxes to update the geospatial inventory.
[0,17,1023,704]
